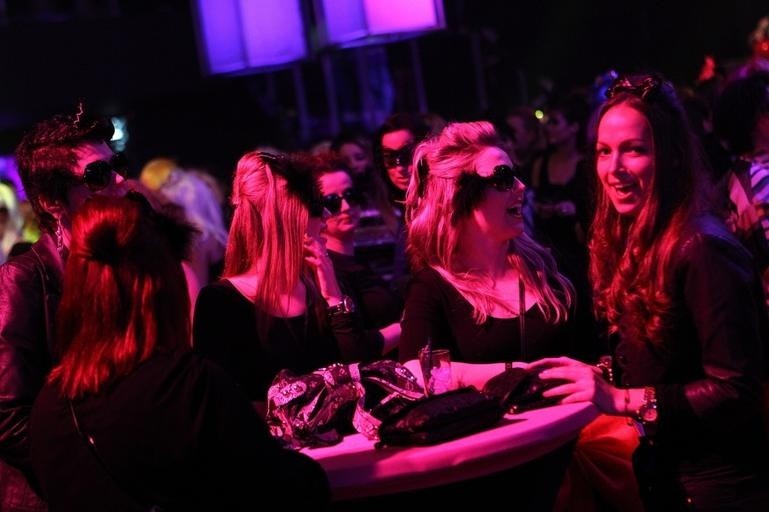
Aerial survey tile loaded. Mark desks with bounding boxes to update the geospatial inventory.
[277,357,599,511]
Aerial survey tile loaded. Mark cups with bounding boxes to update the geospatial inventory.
[418,350,454,398]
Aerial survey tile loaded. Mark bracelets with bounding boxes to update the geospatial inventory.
[624,386,632,417]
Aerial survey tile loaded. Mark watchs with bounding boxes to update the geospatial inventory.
[636,383,659,423]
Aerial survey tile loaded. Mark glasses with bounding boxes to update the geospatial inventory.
[322,186,364,215]
[482,163,523,192]
[70,151,131,193]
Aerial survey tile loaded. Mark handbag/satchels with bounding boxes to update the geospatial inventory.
[481,365,568,412]
[271,357,427,451]
[378,382,500,447]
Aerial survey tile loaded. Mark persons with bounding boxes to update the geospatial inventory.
[0,37,769,363]
[1,108,130,512]
[520,67,769,512]
[191,151,386,402]
[24,177,333,512]
[396,120,592,396]
